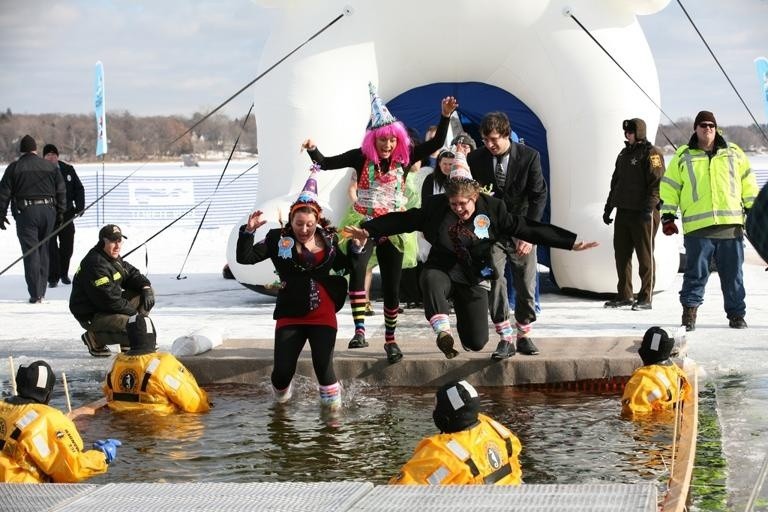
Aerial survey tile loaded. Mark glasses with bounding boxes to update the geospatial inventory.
[699,123,715,129]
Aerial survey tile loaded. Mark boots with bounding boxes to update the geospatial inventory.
[681,305,699,331]
[729,316,748,329]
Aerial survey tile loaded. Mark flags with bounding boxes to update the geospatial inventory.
[93,59,109,161]
[753,53,768,123]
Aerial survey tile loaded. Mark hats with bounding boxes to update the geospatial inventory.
[126,315,156,349]
[16,361,56,403]
[449,140,473,180]
[20,135,37,152]
[367,82,397,129]
[623,118,647,141]
[289,163,324,212]
[639,327,675,363]
[694,111,718,131]
[43,144,59,159]
[100,224,127,241]
[433,379,480,432]
[451,130,477,151]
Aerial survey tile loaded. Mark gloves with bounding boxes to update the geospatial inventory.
[603,204,613,225]
[93,437,122,464]
[55,214,63,227]
[661,214,678,236]
[141,288,155,311]
[0,217,10,230]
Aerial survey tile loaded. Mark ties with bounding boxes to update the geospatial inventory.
[496,155,505,189]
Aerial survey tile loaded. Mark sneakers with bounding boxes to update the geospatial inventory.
[82,330,112,356]
[605,297,634,308]
[633,301,652,310]
[437,330,460,359]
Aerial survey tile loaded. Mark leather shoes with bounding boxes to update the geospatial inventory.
[518,338,541,354]
[30,297,41,302]
[61,277,71,284]
[49,281,57,288]
[492,341,516,359]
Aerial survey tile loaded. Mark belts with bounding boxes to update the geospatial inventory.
[29,199,53,205]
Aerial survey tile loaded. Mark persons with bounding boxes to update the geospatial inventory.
[101,314,215,420]
[236,199,368,408]
[659,111,754,331]
[601,117,665,310]
[298,78,600,366]
[623,326,691,422]
[69,222,156,358]
[43,144,86,288]
[1,356,124,484]
[387,379,526,486]
[1,131,63,306]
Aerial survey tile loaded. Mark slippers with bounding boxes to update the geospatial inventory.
[383,342,403,362]
[347,335,368,347]
